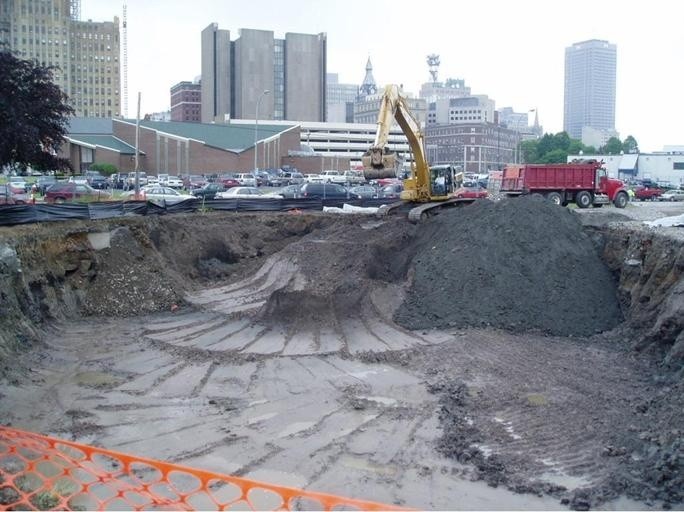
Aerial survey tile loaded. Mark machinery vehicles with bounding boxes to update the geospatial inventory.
[360,82,478,224]
[515,109,535,165]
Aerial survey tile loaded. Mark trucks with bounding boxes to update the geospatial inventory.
[499,159,629,208]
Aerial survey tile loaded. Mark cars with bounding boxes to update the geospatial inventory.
[363,165,503,200]
[623,178,684,202]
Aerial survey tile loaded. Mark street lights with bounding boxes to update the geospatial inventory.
[253,90,270,188]
